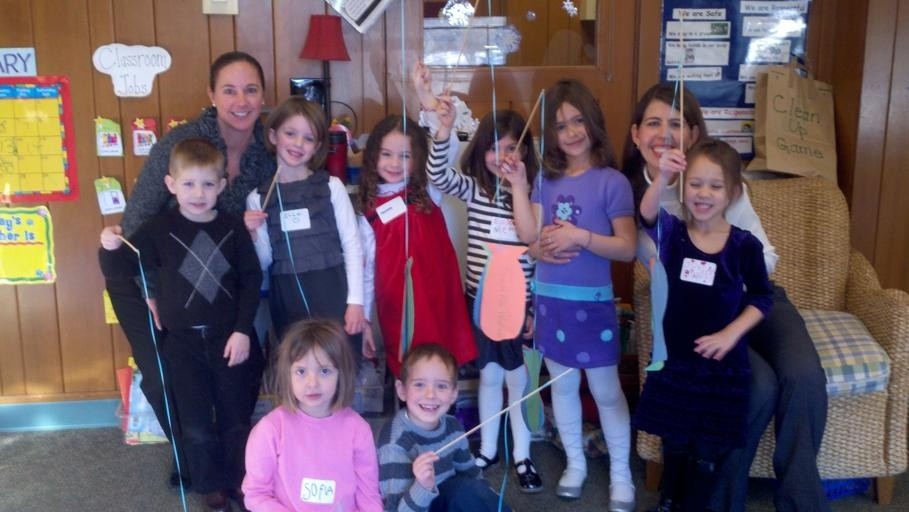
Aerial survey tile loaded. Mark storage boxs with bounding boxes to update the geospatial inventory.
[425,15,510,64]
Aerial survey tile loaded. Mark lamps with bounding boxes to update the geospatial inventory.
[299,12,358,136]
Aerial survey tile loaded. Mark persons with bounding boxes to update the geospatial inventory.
[248,97,363,380]
[639,139,771,511]
[136,137,263,508]
[425,87,541,493]
[501,77,636,511]
[356,62,476,416]
[99,52,274,497]
[377,341,519,511]
[622,80,826,509]
[241,319,382,512]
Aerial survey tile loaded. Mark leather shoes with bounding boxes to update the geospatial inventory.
[168,454,194,497]
[512,458,545,494]
[607,480,637,511]
[556,467,588,499]
[201,487,233,512]
[232,484,252,512]
[471,451,503,475]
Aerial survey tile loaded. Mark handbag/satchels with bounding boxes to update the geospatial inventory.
[747,50,839,189]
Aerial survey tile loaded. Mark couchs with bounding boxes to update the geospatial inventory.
[636,177,903,506]
